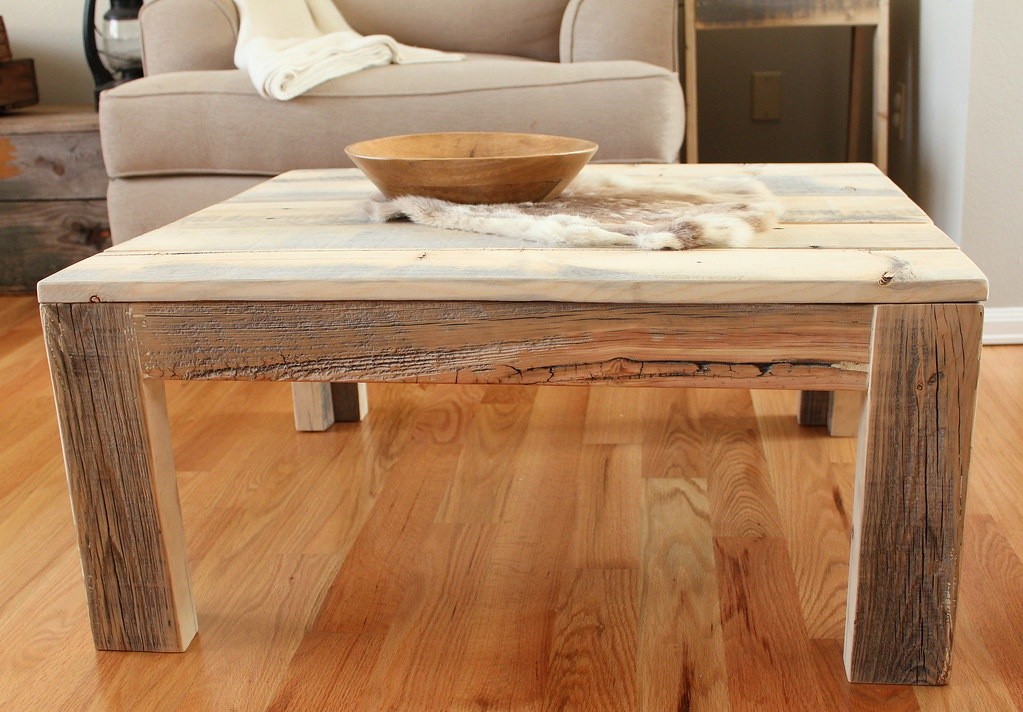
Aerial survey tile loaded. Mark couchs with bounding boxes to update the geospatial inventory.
[101,0,687,430]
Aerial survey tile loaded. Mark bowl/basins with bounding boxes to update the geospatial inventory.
[344,132,599,206]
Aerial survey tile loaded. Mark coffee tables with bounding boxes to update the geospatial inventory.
[37,163,990,685]
